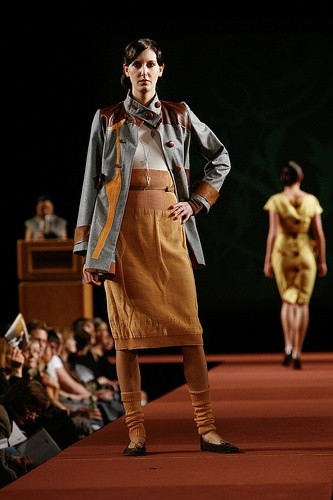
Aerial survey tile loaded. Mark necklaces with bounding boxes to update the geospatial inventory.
[138,132,157,185]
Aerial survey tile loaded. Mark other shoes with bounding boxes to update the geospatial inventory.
[200,435,239,453]
[283,353,292,366]
[123,443,147,456]
[293,359,301,369]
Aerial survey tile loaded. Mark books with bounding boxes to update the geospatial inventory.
[18,428,61,468]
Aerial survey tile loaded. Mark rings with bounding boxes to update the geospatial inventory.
[180,207,183,211]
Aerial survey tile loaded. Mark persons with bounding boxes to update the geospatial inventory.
[74,37,240,456]
[25,197,67,239]
[265,161,326,369]
[0,317,146,488]
